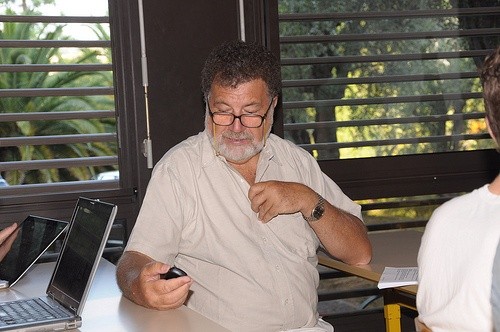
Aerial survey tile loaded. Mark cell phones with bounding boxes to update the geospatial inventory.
[165,267,187,279]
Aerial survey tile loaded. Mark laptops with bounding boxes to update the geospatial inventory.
[0,197,119,332]
[0,214,68,288]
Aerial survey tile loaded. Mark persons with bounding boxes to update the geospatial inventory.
[0,222,17,246]
[416,45,500,332]
[116,40,373,332]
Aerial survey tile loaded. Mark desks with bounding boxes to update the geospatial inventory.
[0,257,233,332]
[311,228,424,332]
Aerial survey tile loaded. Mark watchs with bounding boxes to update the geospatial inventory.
[302,193,326,222]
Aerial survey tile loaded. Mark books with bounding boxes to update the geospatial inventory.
[377,266,418,289]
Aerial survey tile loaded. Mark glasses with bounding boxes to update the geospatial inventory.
[206,93,273,129]
